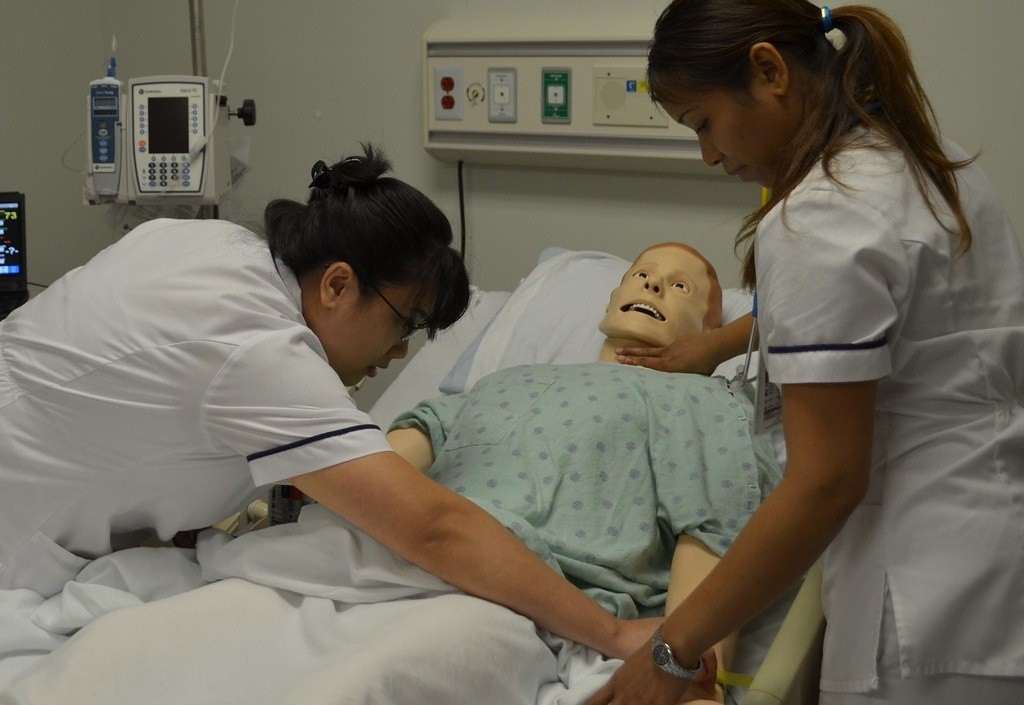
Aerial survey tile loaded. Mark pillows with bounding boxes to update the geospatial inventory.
[434,245,761,395]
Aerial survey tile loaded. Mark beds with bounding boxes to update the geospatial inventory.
[1,283,827,705]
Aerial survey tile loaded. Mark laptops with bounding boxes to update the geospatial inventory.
[0,191,29,321]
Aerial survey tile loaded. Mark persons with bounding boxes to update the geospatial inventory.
[584,0,1024,705]
[0,153,718,705]
[387,242,762,705]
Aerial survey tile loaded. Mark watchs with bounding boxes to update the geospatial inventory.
[652,624,703,680]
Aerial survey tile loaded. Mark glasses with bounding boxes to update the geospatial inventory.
[356,269,424,343]
